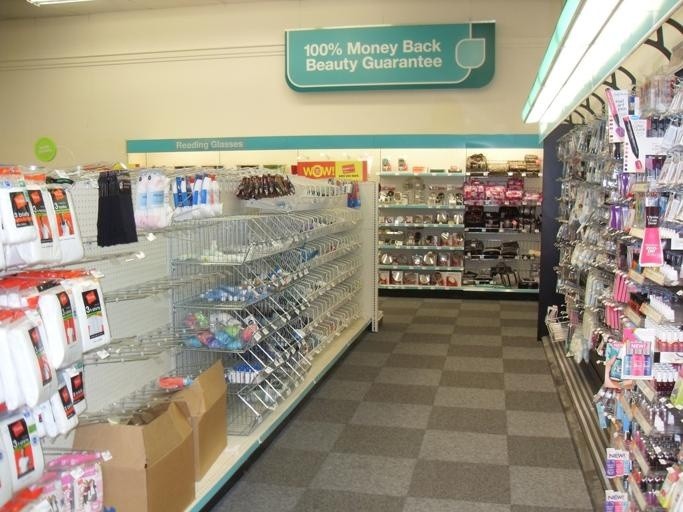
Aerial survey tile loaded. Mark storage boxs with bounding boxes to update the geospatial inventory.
[73,401,196,512]
[143,358,228,481]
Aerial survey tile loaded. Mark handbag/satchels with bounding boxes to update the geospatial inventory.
[465,206,542,233]
[465,240,519,259]
[464,262,520,287]
[463,179,543,200]
[467,154,541,173]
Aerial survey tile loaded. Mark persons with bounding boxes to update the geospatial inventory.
[59,212,72,236]
[67,319,76,343]
[39,215,50,239]
[18,447,32,475]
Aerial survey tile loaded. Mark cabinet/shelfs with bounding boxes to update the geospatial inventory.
[376,171,541,294]
[0,164,384,512]
